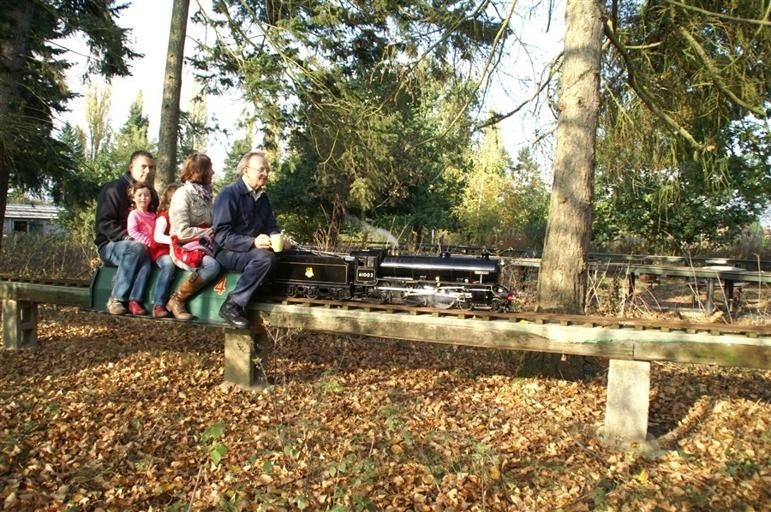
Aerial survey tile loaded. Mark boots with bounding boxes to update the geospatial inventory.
[166,270,206,320]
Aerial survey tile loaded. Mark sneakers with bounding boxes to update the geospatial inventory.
[128,301,146,315]
[106,296,127,314]
[152,304,169,318]
[219,296,250,329]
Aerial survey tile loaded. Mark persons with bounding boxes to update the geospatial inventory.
[148,182,181,318]
[167,152,220,320]
[209,152,293,329]
[92,149,159,318]
[124,182,155,316]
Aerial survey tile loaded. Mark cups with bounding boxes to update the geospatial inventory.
[268,234,284,253]
